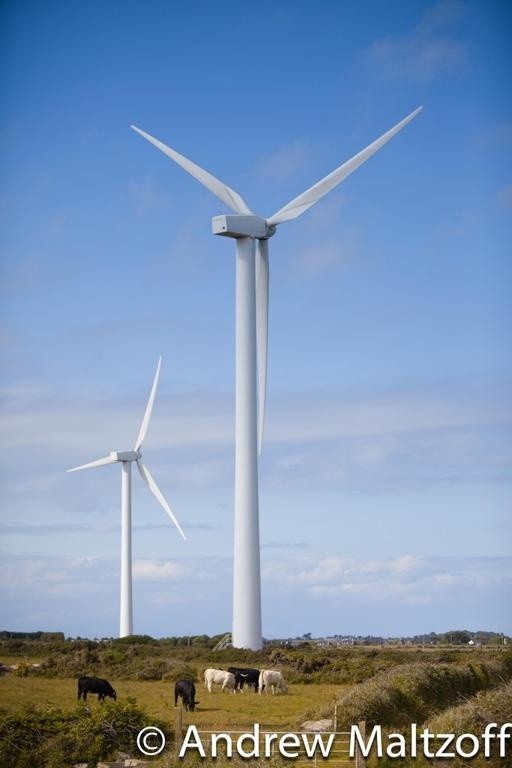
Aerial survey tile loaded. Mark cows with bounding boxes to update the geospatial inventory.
[173,679,201,713]
[203,668,237,694]
[257,669,288,696]
[227,666,266,694]
[78,675,118,705]
